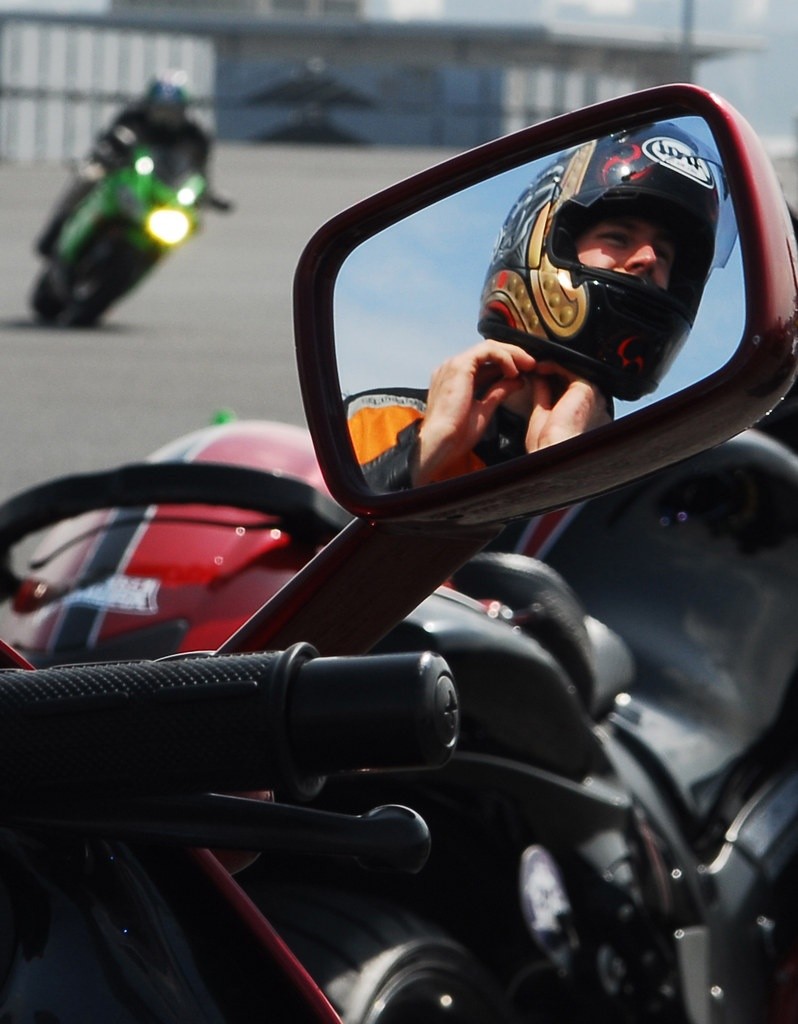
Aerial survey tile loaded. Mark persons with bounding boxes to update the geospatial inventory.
[339,117,758,500]
[37,69,215,268]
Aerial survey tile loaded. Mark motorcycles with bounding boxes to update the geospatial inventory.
[0,87,798,1022]
[26,125,236,330]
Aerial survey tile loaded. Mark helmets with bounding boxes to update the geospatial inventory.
[477,124,718,403]
[144,77,187,117]
